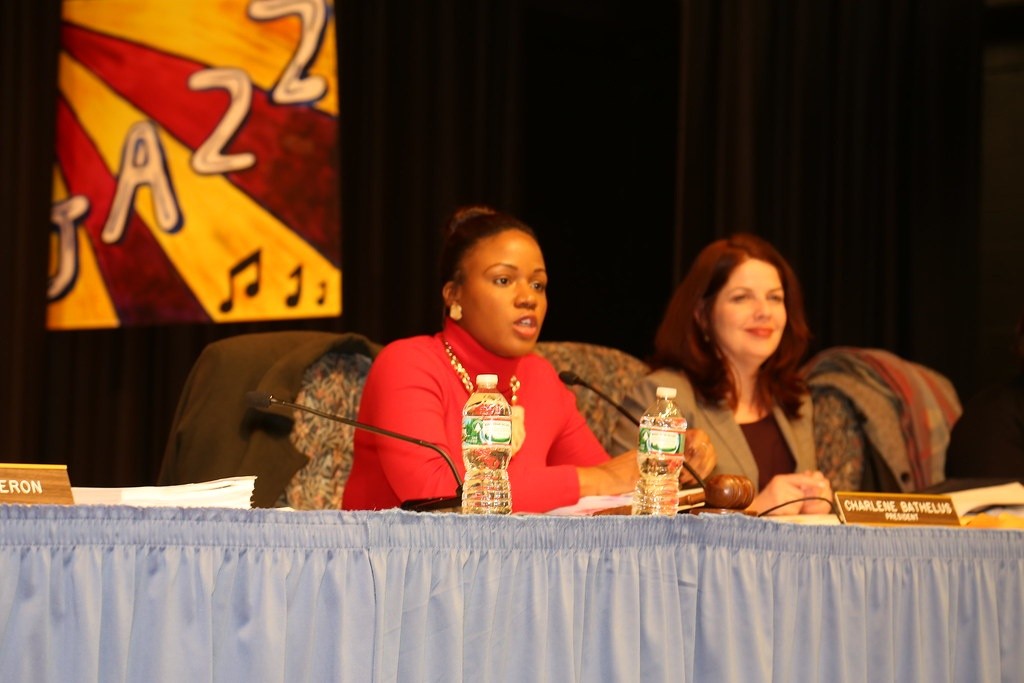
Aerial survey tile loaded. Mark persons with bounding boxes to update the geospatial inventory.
[341,205,717,514]
[609,232,833,516]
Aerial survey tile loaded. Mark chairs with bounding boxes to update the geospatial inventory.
[177,332,388,509]
[532,341,647,456]
[807,373,877,493]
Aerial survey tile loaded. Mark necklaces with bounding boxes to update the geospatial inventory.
[442,342,520,409]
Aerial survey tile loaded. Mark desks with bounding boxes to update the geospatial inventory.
[0,483,1024,683]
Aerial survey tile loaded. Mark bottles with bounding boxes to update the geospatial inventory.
[632,386,686,516]
[461,375,513,515]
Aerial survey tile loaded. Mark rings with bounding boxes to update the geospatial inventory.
[701,440,710,447]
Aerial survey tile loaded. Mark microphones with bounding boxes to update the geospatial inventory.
[249,393,465,513]
[558,371,706,488]
